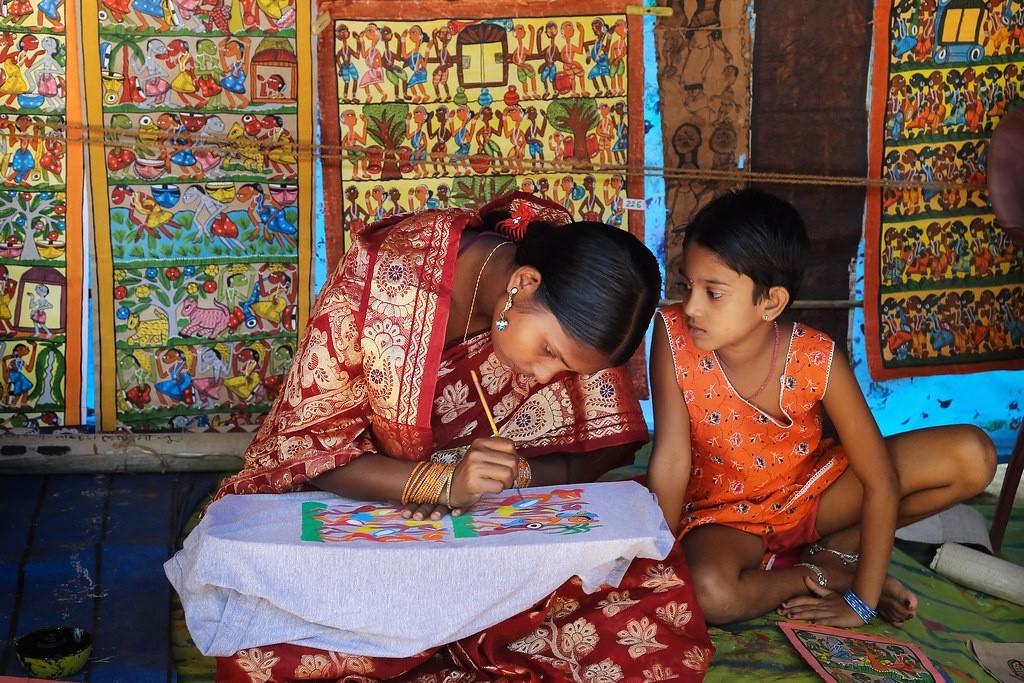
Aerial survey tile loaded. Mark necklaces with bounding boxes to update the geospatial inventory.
[463,241,513,341]
[713,321,780,404]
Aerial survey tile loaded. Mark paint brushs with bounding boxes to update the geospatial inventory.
[471,369,525,502]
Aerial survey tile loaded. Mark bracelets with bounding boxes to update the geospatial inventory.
[842,590,879,626]
[511,456,532,489]
[402,460,454,511]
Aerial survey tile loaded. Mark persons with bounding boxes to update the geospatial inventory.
[199,192,715,683]
[640,189,999,628]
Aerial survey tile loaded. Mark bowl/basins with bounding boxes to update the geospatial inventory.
[15,627,93,679]
